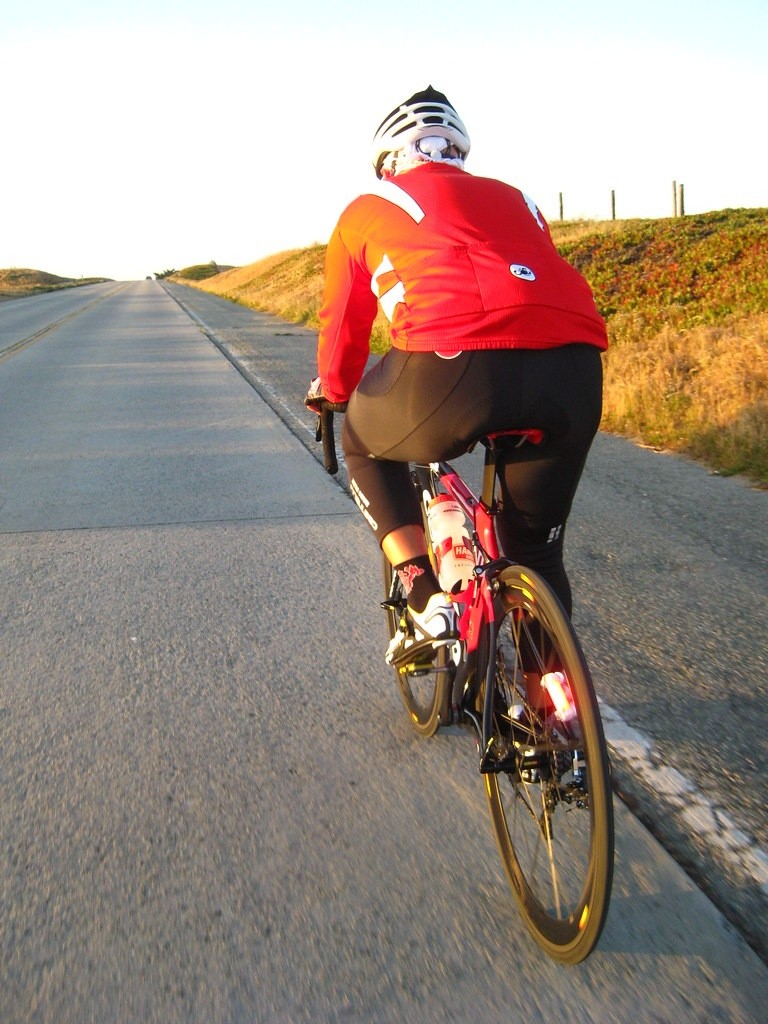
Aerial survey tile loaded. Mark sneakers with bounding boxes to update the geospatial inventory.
[385,592,461,666]
[508,704,540,784]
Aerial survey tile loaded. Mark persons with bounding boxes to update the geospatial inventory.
[302,88,608,798]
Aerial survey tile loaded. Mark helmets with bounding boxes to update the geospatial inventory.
[370,84,471,180]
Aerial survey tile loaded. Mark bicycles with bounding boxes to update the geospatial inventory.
[308,397,622,968]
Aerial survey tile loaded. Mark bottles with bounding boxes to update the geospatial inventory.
[426,491,477,591]
